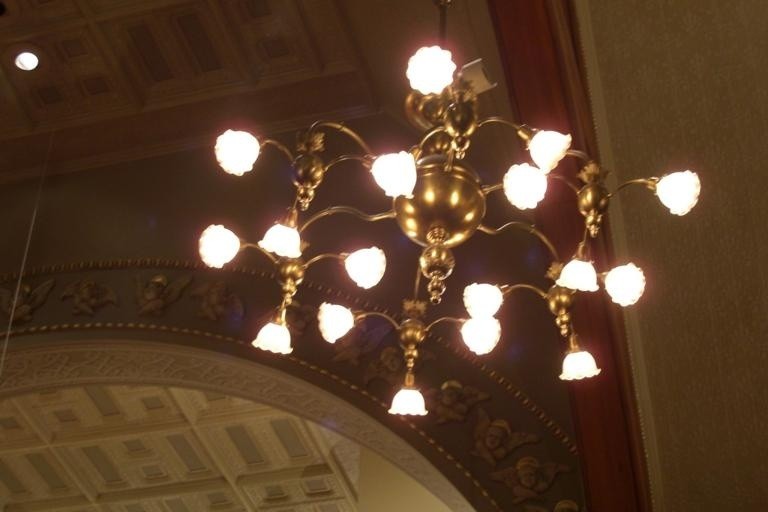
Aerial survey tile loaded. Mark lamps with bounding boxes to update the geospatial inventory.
[196,0,700,419]
